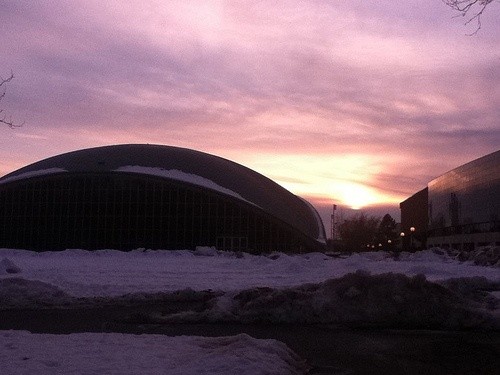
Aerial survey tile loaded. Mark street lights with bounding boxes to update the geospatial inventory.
[400,232,405,251]
[410,227,416,252]
